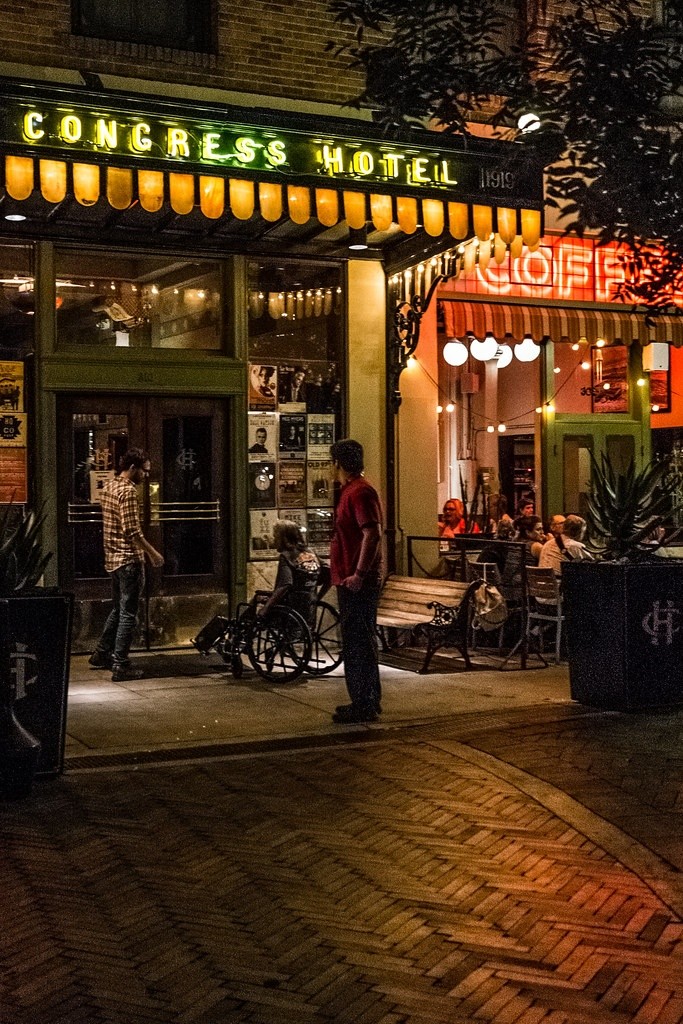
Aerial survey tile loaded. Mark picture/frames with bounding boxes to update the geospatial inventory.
[590,338,673,414]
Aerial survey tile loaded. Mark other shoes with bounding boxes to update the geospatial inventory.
[111,663,145,681]
[332,702,383,723]
[88,652,112,669]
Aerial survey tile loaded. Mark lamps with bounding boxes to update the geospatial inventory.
[491,340,511,369]
[111,280,117,290]
[514,338,539,361]
[131,283,137,291]
[442,338,469,367]
[257,283,345,300]
[470,333,497,362]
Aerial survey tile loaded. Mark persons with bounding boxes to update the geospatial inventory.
[437,498,534,540]
[194,518,329,652]
[329,440,385,724]
[279,367,340,413]
[249,428,268,453]
[284,425,301,448]
[88,447,164,681]
[476,515,596,653]
[253,365,275,397]
[259,512,274,549]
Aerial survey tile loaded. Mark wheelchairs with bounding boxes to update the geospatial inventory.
[190,565,343,683]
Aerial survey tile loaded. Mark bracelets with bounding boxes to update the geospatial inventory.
[354,568,366,578]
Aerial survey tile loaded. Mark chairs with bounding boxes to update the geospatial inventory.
[524,565,565,662]
[350,572,486,673]
[443,557,477,582]
[471,561,522,650]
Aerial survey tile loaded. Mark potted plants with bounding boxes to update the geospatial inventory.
[554,448,683,712]
[1,506,77,778]
